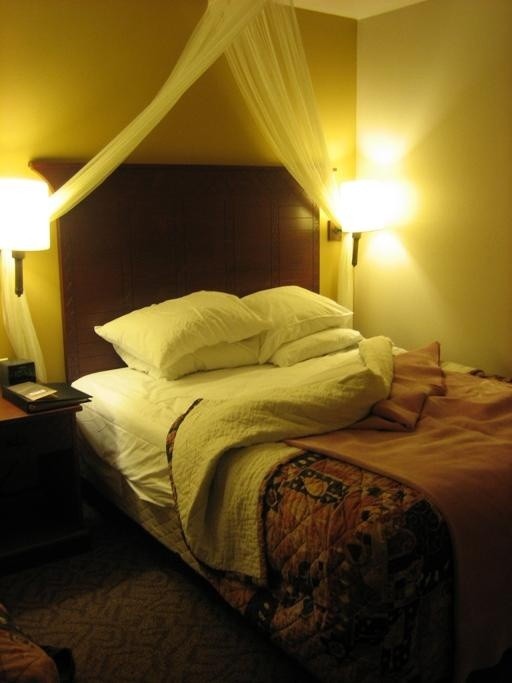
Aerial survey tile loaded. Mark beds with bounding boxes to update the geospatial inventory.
[29,158,512,683]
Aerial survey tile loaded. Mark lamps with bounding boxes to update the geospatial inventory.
[327,180,373,267]
[0,178,50,297]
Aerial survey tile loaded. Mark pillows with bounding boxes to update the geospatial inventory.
[270,327,364,369]
[91,290,272,366]
[239,284,353,363]
[112,337,261,383]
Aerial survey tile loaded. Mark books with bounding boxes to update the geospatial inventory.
[2,383,92,413]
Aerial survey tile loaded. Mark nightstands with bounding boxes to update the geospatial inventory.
[0,385,95,576]
